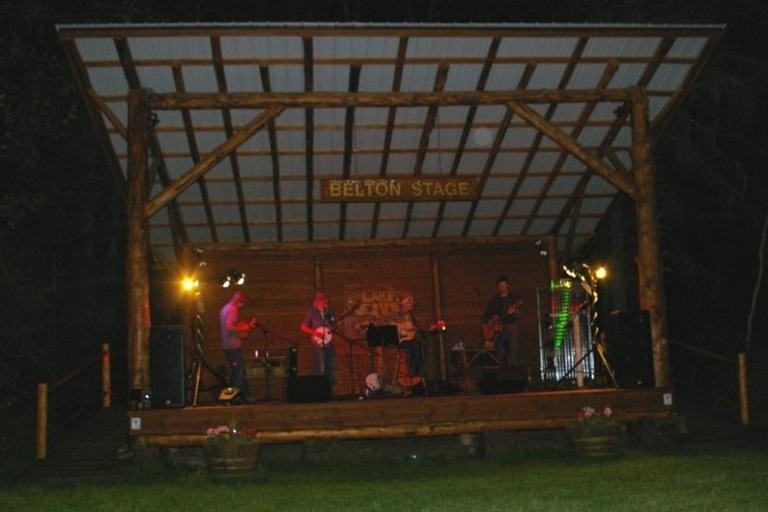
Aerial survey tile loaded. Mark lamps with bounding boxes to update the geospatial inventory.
[561,257,586,279]
[196,268,247,291]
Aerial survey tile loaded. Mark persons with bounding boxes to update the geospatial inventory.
[482,275,528,346]
[381,291,439,377]
[299,291,344,388]
[220,289,259,405]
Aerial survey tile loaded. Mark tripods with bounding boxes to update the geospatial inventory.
[185,283,225,408]
[554,294,619,389]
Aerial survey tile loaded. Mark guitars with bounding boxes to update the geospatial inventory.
[482,299,522,341]
[397,321,445,343]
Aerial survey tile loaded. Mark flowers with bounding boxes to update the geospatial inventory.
[200,425,261,446]
[565,406,619,439]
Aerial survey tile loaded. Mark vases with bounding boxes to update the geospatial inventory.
[568,435,619,462]
[206,448,262,484]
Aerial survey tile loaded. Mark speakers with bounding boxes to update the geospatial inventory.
[479,367,528,395]
[608,309,654,388]
[368,325,398,348]
[287,376,330,403]
[150,325,185,408]
